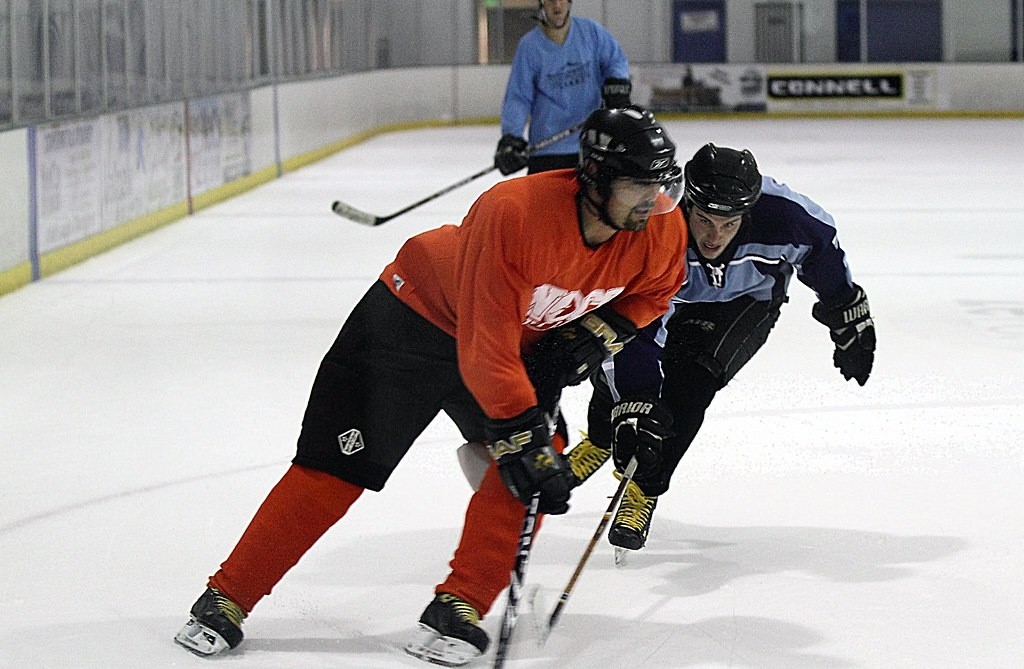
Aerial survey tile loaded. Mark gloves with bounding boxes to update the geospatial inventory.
[610,399,673,478]
[536,305,639,390]
[601,78,632,109]
[494,132,531,176]
[812,285,875,387]
[483,411,576,516]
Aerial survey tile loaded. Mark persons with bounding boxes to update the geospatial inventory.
[186,100,692,658]
[495,0,633,176]
[562,143,876,553]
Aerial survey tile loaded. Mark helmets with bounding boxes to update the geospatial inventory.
[683,144,763,217]
[575,106,675,198]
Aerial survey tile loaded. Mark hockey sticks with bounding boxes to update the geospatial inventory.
[490,384,565,669]
[330,121,585,228]
[524,455,640,657]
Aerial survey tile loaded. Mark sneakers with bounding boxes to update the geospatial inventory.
[404,591,490,667]
[561,431,612,491]
[608,471,658,564]
[174,585,245,657]
